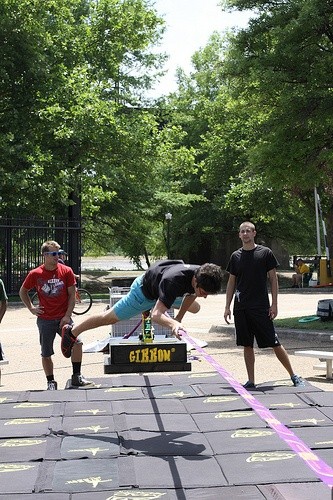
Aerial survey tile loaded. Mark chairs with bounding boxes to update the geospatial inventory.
[297,267,314,288]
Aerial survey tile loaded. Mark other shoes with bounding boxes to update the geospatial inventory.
[46,380,56,391]
[290,374,304,387]
[70,374,94,389]
[242,381,256,391]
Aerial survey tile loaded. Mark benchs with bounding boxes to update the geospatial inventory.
[294,350,333,380]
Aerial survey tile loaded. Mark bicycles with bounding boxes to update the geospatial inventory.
[30,275,93,315]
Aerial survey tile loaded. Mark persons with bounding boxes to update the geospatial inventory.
[288,255,331,288]
[0,279,8,389]
[223,221,305,392]
[57,248,80,279]
[61,259,223,359]
[19,240,94,390]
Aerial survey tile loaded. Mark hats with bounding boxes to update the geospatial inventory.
[57,249,67,256]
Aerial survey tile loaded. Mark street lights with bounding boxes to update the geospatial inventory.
[165,213,172,260]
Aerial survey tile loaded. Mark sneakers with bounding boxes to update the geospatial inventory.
[60,324,77,358]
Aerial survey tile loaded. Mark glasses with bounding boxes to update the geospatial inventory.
[45,252,61,256]
